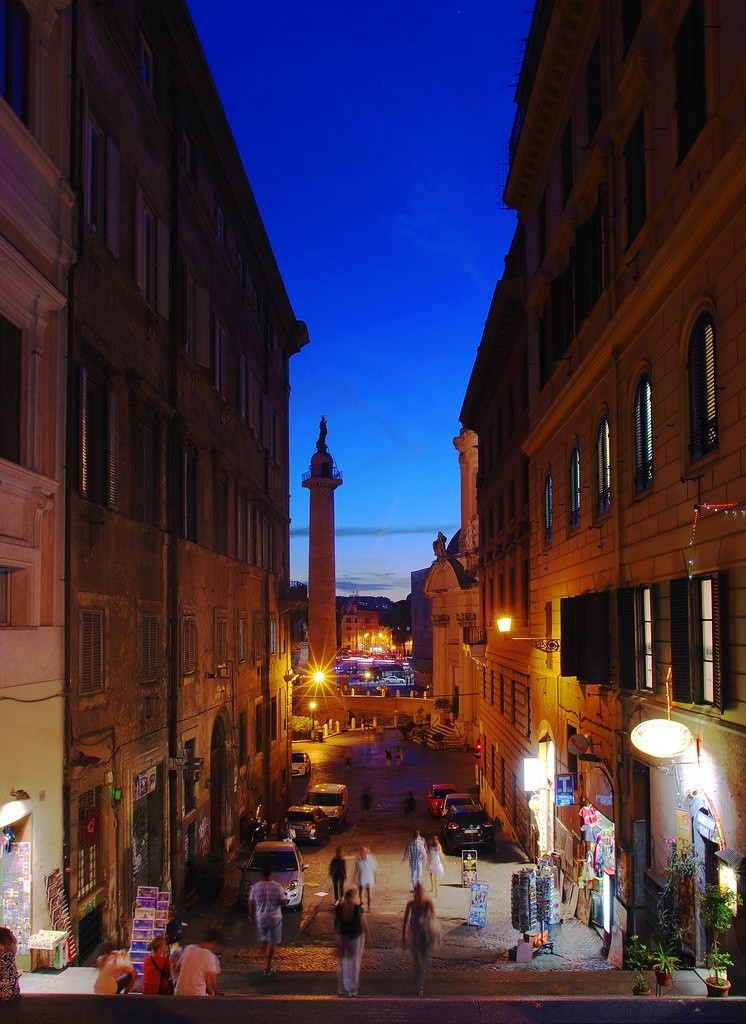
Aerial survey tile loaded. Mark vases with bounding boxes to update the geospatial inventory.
[652,956,675,965]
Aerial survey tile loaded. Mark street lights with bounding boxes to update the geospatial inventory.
[364,673,371,690]
[310,701,318,730]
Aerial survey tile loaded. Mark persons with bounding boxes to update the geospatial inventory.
[0,926,20,1000]
[171,929,223,997]
[351,846,380,912]
[328,847,346,905]
[359,787,372,823]
[403,829,428,892]
[425,835,445,893]
[139,936,174,995]
[332,888,372,999]
[276,815,290,840]
[383,747,393,766]
[247,867,288,978]
[93,944,137,995]
[395,744,402,767]
[400,883,437,997]
[402,790,417,831]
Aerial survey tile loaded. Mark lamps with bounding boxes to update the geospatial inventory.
[496,618,561,654]
[657,761,696,775]
[538,732,552,743]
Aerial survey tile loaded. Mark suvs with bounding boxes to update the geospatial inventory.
[302,783,349,828]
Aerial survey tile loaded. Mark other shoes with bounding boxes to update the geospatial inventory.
[359,901,364,907]
[366,901,371,909]
[263,967,277,977]
[333,899,338,906]
[410,890,415,893]
[338,989,347,997]
[348,990,358,997]
[340,898,344,904]
[416,987,423,997]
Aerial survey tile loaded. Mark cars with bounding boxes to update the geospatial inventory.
[236,840,309,911]
[440,811,495,856]
[278,804,330,846]
[439,793,479,818]
[426,784,459,817]
[383,675,406,684]
[292,752,312,777]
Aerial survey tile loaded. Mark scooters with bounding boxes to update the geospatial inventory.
[247,810,268,850]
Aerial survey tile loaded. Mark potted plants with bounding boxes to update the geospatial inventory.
[416,729,431,752]
[431,733,444,751]
[651,942,682,986]
[398,721,416,741]
[433,697,449,725]
[693,882,744,997]
[624,934,653,996]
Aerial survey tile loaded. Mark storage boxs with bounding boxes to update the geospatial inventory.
[593,877,603,893]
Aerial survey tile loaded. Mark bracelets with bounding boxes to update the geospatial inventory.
[248,916,252,918]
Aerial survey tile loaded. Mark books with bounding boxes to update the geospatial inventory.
[45,868,77,959]
[129,885,169,991]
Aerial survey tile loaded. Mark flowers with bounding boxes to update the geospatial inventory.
[654,838,704,958]
